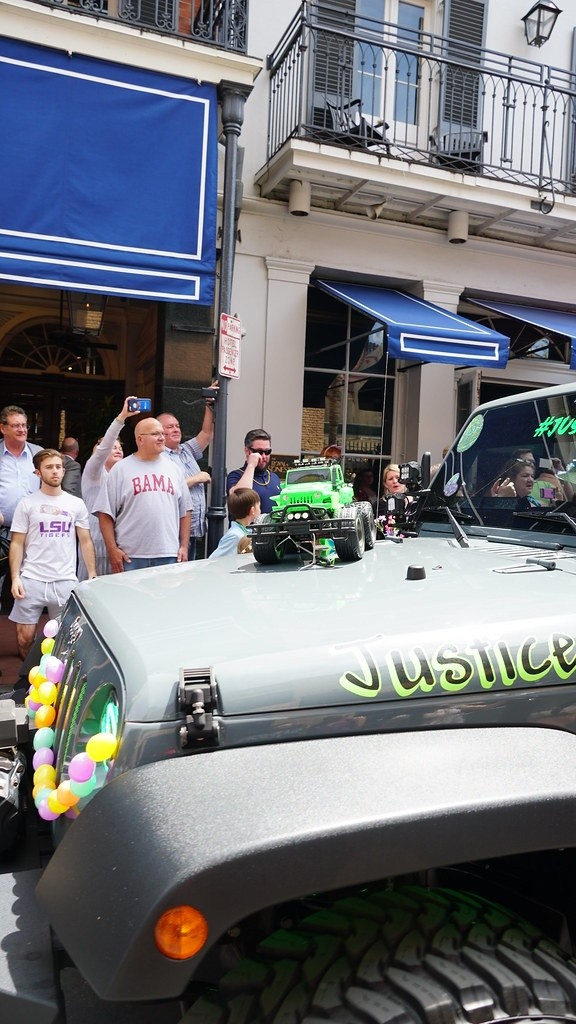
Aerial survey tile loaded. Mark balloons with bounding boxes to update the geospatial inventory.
[24,620,118,820]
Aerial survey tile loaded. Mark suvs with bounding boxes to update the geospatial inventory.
[246,457,376,565]
[35,382,575,1023]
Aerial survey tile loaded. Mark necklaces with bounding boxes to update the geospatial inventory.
[238,469,271,486]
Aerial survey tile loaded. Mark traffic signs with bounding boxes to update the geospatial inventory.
[218,313,243,381]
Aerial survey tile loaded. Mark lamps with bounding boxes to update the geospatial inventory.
[520,0,563,46]
[64,289,108,337]
[289,179,313,216]
[449,210,470,243]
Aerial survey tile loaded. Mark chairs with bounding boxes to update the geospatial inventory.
[427,126,489,174]
[321,92,390,153]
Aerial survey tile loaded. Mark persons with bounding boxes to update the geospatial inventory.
[155,379,220,560]
[209,488,261,558]
[0,404,44,614]
[320,443,343,460]
[372,464,413,540]
[92,417,194,574]
[226,429,283,514]
[7,448,97,662]
[59,437,83,498]
[488,448,574,508]
[76,396,141,584]
[430,445,463,497]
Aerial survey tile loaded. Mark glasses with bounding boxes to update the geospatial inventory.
[6,423,30,430]
[140,431,165,438]
[512,459,531,468]
[248,447,272,456]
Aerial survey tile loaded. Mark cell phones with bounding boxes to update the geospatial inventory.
[540,458,553,469]
[202,387,218,399]
[128,397,151,412]
[540,488,556,499]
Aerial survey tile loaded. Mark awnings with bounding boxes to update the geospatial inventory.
[463,296,576,371]
[313,279,509,370]
